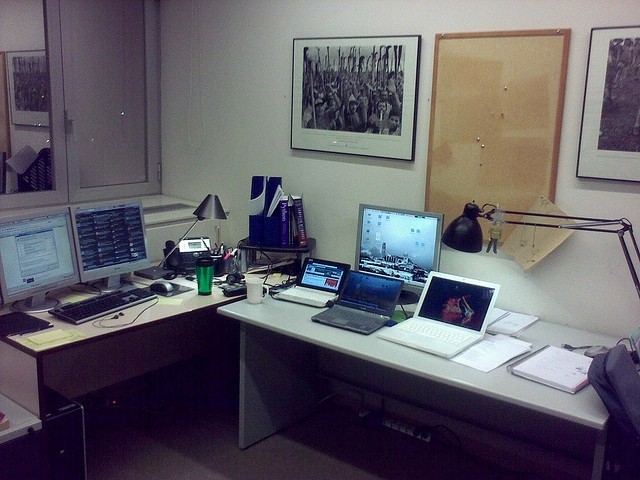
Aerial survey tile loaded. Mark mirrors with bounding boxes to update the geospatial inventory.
[0,0,53,195]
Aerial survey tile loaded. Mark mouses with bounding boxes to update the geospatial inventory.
[150,281,175,293]
[584,346,609,357]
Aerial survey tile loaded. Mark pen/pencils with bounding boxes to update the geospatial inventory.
[201,238,230,261]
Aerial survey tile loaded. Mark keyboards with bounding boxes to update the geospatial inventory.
[48,284,158,325]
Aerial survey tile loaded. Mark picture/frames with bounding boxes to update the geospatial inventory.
[576,25,640,183]
[6,50,49,127]
[424,28,570,245]
[290,34,422,162]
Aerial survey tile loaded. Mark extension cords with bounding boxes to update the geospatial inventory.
[359,407,433,442]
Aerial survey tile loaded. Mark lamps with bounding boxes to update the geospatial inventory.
[134,193,228,280]
[441,200,639,302]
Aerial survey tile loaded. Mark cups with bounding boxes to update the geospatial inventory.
[247,279,269,304]
[195,258,215,295]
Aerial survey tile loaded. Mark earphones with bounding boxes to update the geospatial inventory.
[119,312,125,316]
[112,314,119,319]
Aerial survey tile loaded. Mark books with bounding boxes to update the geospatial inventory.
[504,344,593,395]
[290,194,307,247]
[279,195,288,247]
[287,203,294,248]
[486,307,540,339]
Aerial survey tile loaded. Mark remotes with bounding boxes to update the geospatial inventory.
[224,283,247,297]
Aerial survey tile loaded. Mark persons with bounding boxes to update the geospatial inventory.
[485,221,502,254]
[301,46,404,136]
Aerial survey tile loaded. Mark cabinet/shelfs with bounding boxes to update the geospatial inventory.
[59,0,161,202]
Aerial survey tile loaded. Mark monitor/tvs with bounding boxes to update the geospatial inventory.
[0,205,80,314]
[68,197,151,294]
[354,203,444,305]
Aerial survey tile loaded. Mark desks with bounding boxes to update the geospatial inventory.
[217,283,640,480]
[0,248,296,420]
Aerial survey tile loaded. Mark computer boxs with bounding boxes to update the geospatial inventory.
[13,385,87,476]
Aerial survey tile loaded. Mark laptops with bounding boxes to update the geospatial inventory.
[273,256,351,308]
[311,269,405,335]
[377,270,501,359]
